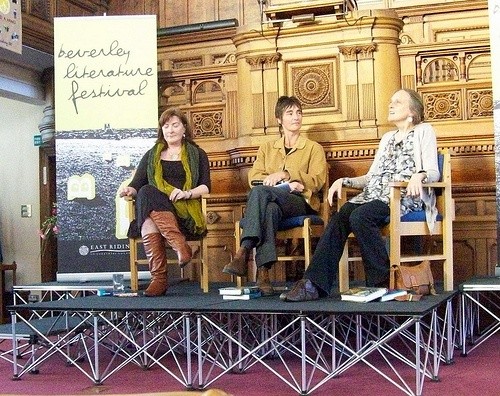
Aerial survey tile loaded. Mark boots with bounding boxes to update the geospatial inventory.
[149,210,193,268]
[142,233,167,297]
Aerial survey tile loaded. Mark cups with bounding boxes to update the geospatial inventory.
[112,274,124,295]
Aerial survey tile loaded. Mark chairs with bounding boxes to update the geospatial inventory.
[337,149,455,292]
[0,262,30,324]
[235,162,330,288]
[124,194,210,293]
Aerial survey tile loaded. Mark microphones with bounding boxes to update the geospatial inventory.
[251,180,282,186]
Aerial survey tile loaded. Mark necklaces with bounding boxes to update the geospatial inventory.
[166,148,181,160]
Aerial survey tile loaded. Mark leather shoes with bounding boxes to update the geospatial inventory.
[257,268,275,296]
[222,248,250,278]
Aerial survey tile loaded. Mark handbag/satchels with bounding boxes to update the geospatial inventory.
[389,260,435,301]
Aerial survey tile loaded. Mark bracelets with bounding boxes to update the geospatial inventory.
[345,176,351,188]
[188,191,192,200]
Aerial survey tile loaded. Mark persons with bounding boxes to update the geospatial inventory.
[222,95,328,297]
[118,108,211,297]
[279,88,441,301]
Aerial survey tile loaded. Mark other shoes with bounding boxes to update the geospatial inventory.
[278,279,319,301]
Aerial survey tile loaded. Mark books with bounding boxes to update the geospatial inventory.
[113,290,145,297]
[219,286,259,295]
[96,289,125,296]
[340,287,389,304]
[223,292,261,301]
[382,287,409,302]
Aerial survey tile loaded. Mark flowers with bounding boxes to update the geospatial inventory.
[37,202,57,258]
